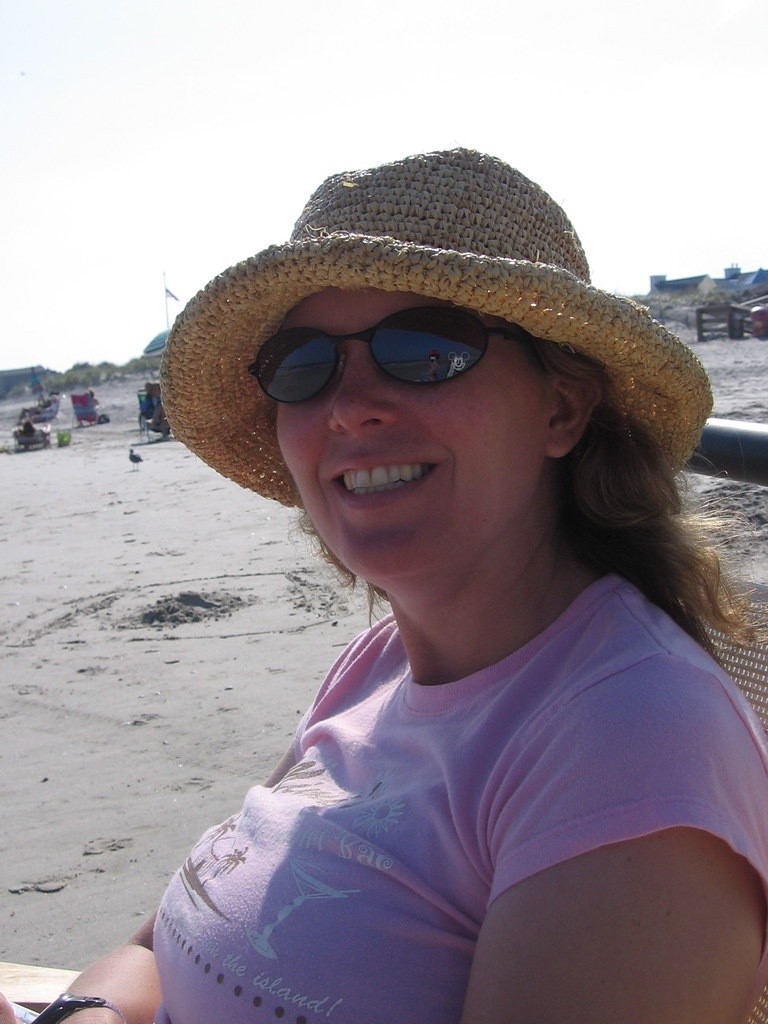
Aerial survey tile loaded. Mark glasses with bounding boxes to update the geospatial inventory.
[248,305,532,404]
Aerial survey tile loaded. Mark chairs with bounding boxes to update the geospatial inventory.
[12,429,51,451]
[137,388,166,441]
[32,392,60,422]
[71,392,99,425]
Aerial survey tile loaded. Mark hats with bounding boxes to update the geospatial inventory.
[160,148,715,511]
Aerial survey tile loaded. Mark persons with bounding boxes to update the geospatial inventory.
[17,390,101,436]
[0,147,768,1024]
[139,382,158,435]
[427,350,440,368]
[145,384,172,442]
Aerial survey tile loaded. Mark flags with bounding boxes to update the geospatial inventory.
[165,289,179,303]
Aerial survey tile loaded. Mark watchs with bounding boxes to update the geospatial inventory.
[30,993,126,1024]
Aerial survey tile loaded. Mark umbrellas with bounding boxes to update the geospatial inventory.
[30,368,43,406]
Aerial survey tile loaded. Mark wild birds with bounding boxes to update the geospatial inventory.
[128,449,144,471]
